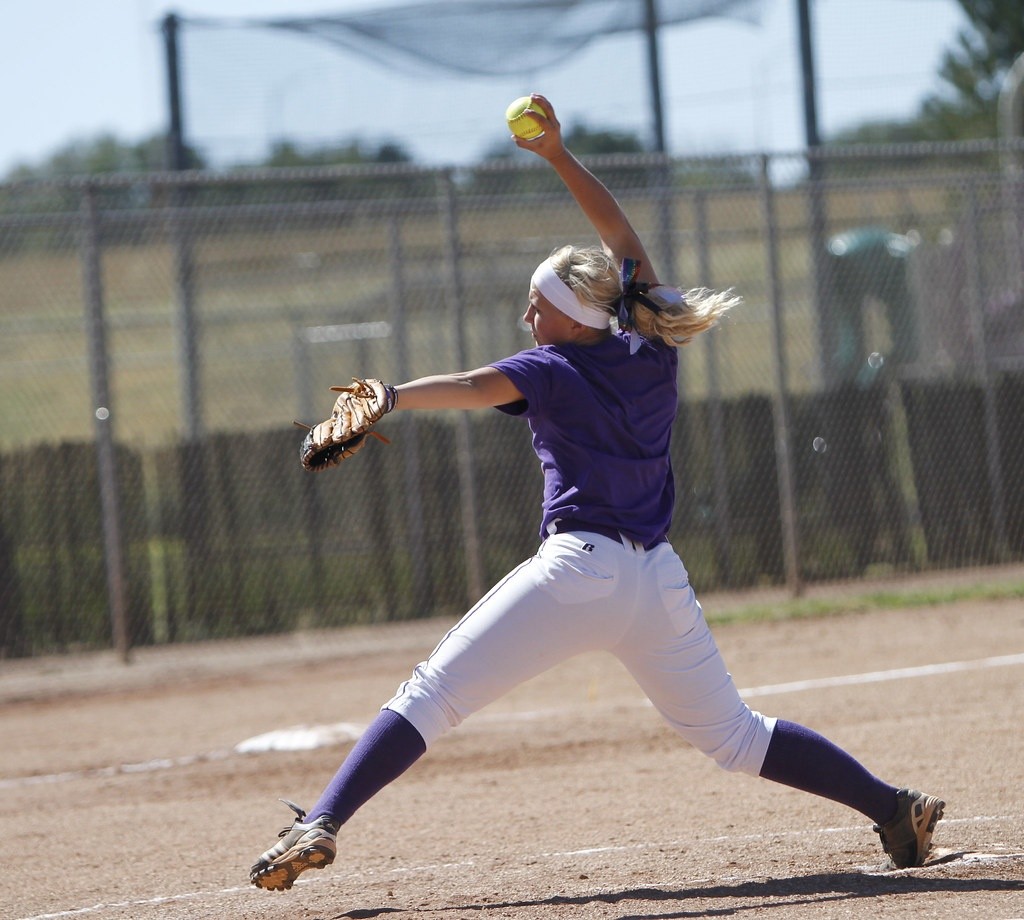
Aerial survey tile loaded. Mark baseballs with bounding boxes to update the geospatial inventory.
[505,95,547,140]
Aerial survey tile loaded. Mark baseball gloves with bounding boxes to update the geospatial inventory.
[298,379,399,472]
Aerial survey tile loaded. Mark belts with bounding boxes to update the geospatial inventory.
[542,519,669,552]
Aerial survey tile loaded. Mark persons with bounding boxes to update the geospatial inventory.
[249,91,947,889]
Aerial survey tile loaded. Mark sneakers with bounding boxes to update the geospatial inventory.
[873,788,946,870]
[249,798,340,892]
